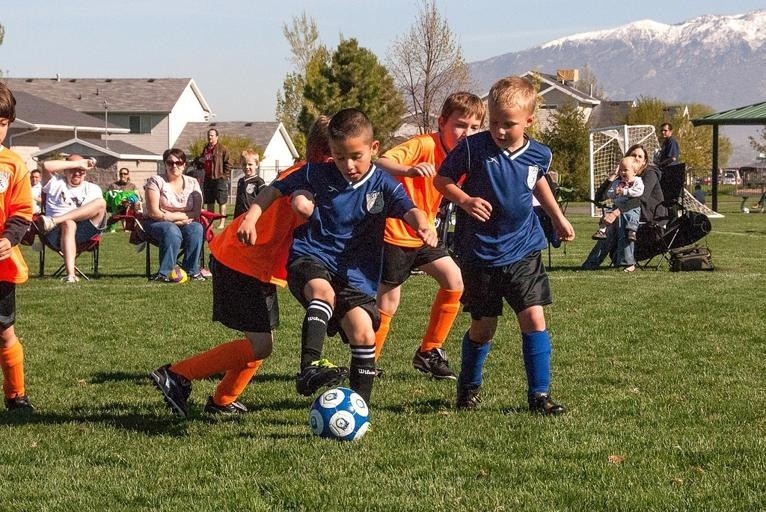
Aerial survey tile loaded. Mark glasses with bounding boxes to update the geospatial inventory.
[161,159,185,167]
[118,172,128,175]
[660,129,668,132]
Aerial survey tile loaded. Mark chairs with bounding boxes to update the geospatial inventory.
[107,197,141,233]
[32,182,104,281]
[132,203,229,280]
[605,162,690,271]
[534,169,571,272]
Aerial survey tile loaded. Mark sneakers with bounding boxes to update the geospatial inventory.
[411,345,457,381]
[2,395,38,416]
[526,392,565,416]
[202,394,253,416]
[149,361,191,422]
[36,213,55,233]
[591,230,606,240]
[59,274,81,285]
[294,360,350,397]
[627,230,637,242]
[189,271,206,282]
[455,386,483,412]
[150,273,171,283]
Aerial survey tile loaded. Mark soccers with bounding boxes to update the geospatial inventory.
[742,207,749,213]
[309,387,369,440]
[170,268,187,283]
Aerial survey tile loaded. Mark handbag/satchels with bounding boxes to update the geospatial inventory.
[670,246,716,274]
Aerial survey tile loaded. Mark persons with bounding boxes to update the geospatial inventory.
[581,123,681,272]
[752,191,766,213]
[29,128,266,284]
[0,82,36,415]
[692,184,706,203]
[149,75,576,424]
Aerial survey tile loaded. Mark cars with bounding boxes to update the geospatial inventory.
[694,166,766,191]
